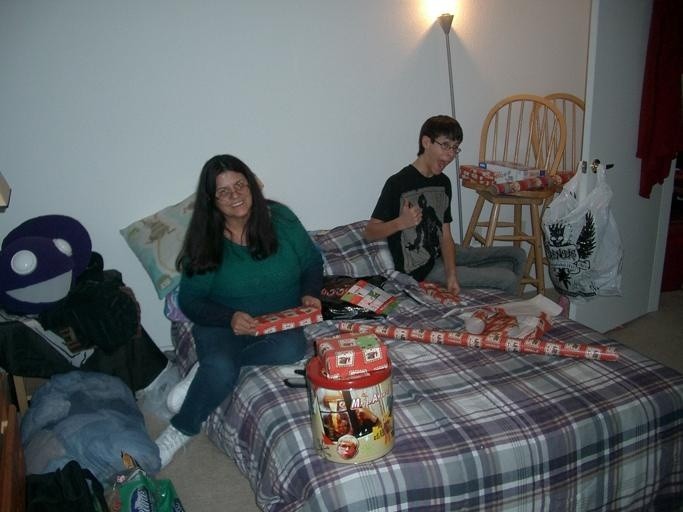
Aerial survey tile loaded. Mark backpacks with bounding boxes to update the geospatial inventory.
[25,459,109,512]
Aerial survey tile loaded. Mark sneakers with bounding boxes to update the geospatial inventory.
[165,360,201,415]
[151,422,202,472]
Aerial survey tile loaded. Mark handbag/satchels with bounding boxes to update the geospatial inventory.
[538,161,626,298]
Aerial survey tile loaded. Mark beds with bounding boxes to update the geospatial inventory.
[171,225,683,512]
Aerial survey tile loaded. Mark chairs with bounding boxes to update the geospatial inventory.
[459,92,565,294]
[533,93,584,183]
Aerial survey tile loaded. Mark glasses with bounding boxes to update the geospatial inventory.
[431,137,463,156]
[211,181,250,201]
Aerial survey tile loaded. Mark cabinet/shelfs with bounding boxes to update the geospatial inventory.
[0,372,24,511]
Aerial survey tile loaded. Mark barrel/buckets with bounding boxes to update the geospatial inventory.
[306,355,396,465]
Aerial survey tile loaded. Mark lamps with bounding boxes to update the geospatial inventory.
[437,13,465,246]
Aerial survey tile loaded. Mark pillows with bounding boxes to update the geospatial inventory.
[118,193,197,302]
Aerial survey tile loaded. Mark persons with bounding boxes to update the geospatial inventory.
[362,116,528,295]
[152,153,325,467]
